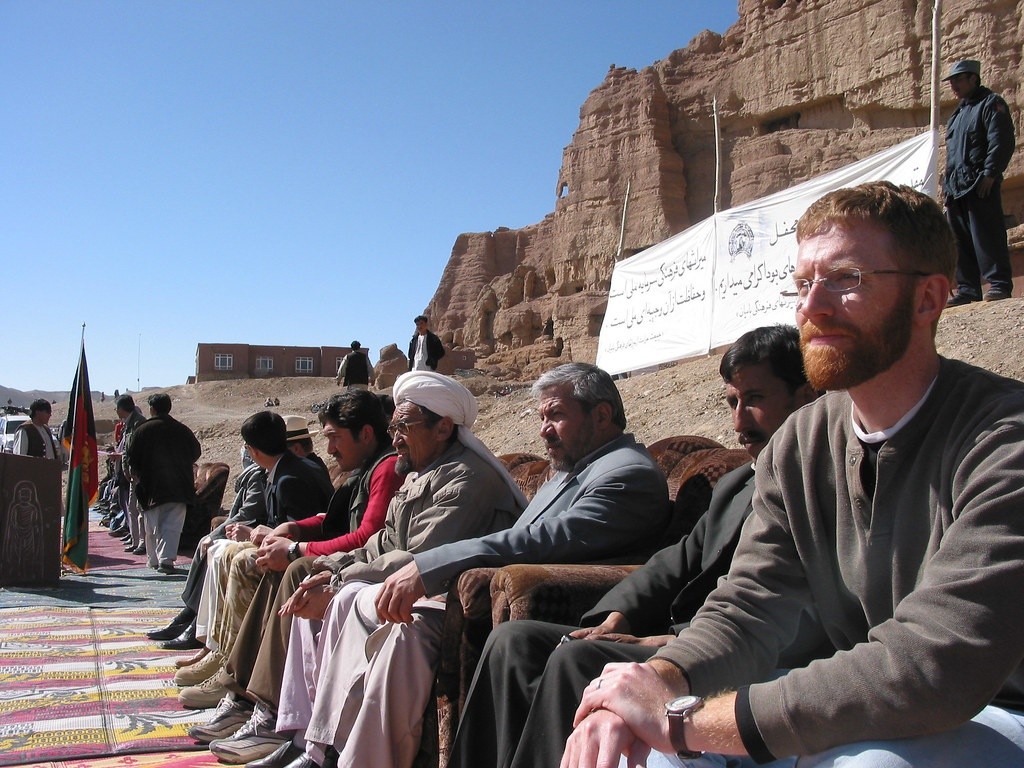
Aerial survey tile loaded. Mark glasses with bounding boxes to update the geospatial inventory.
[387,417,439,439]
[779,266,931,308]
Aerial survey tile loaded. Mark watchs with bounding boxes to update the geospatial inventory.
[663,695,706,759]
[287,541,301,562]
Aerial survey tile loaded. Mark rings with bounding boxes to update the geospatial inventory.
[596,679,602,689]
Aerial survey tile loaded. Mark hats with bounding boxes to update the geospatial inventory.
[283,415,320,440]
[941,59,981,82]
[30,398,51,411]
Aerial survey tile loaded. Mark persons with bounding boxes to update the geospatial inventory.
[939,60,1017,308]
[408,315,445,373]
[56,419,69,464]
[12,399,66,518]
[559,181,1024,767]
[444,326,827,768]
[280,361,669,768]
[336,341,376,392]
[146,370,530,768]
[94,394,202,574]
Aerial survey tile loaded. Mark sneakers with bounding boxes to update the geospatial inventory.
[177,671,234,709]
[209,701,290,763]
[189,692,254,743]
[174,652,224,687]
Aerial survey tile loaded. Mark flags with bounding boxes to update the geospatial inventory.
[61,336,99,574]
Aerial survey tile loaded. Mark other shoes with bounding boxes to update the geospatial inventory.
[152,565,158,570]
[92,505,144,555]
[157,563,175,574]
[176,648,208,669]
[945,295,981,306]
[983,291,1011,300]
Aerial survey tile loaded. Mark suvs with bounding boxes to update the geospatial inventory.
[0,414,32,454]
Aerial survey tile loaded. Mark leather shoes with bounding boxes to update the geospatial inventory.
[161,624,206,649]
[281,747,340,768]
[146,617,189,639]
[246,741,304,768]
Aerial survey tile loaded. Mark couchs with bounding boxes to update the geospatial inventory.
[179,435,752,767]
